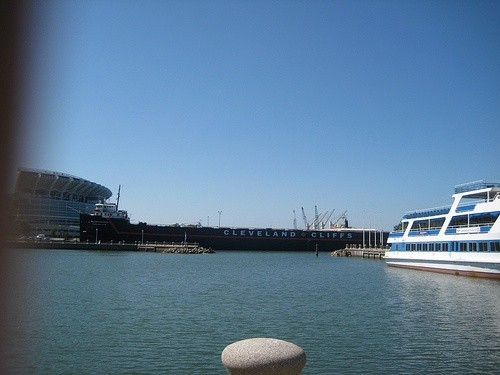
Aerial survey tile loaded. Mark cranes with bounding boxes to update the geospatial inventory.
[293,204,352,230]
[96,184,127,219]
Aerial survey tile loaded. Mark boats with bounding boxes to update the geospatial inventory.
[384,180,500,279]
